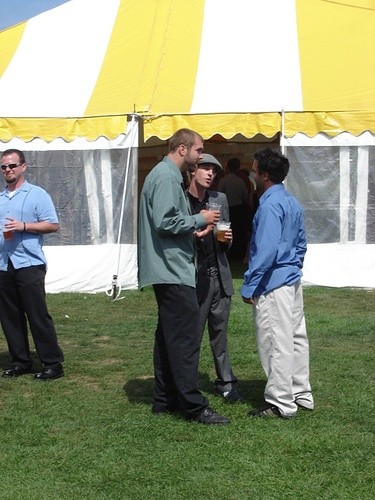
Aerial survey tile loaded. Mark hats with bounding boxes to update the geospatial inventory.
[191,153,223,173]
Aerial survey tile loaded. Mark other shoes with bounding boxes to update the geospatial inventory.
[195,407,231,425]
[248,404,281,419]
[215,390,244,402]
[153,400,173,412]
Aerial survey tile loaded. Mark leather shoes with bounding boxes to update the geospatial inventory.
[2,366,33,377]
[34,368,64,381]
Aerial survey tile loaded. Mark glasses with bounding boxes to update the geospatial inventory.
[1,162,23,170]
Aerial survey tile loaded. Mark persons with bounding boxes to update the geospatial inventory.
[0,148,64,380]
[217,157,248,276]
[238,168,254,267]
[184,153,239,404]
[240,148,314,419]
[139,127,231,425]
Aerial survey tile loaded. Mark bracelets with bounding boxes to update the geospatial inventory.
[23,222,26,231]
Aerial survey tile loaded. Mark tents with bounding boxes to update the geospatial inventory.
[0,0,375,294]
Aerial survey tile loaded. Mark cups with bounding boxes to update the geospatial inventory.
[206,202,222,223]
[1,220,13,240]
[216,221,231,242]
